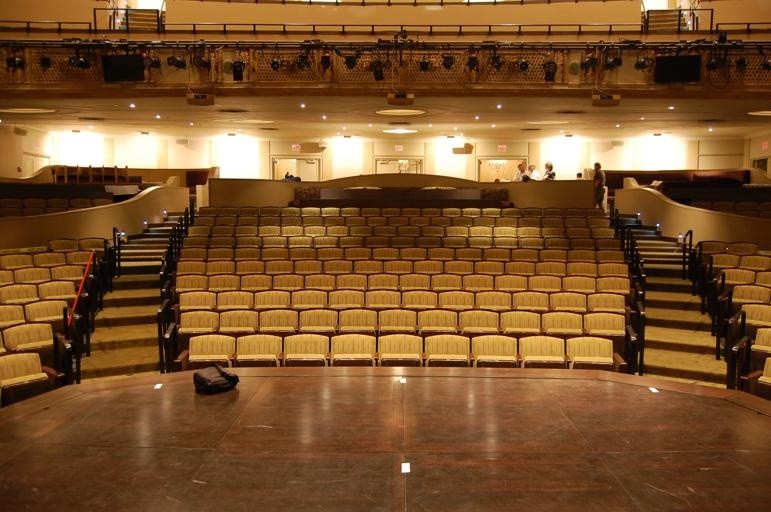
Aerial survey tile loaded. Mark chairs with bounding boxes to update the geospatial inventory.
[693,198,770,394]
[1,196,114,401]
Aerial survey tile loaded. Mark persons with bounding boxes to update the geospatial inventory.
[575,173,584,180]
[592,163,607,209]
[512,161,556,183]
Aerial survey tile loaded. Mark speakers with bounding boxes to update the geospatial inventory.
[101,54,146,82]
[654,54,702,83]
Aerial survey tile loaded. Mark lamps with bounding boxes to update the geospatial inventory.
[580,45,656,70]
[705,51,771,73]
[5,48,93,71]
[146,48,560,83]
